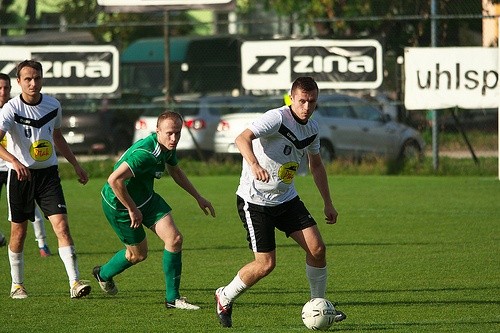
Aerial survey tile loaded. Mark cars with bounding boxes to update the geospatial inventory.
[130,91,230,160]
[212,92,426,164]
[60,99,132,156]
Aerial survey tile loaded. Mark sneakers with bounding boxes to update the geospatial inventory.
[92,266,118,296]
[333,302,346,322]
[39,245,51,257]
[70,279,91,299]
[214,287,233,327]
[8,284,29,299]
[0,232,7,247]
[164,296,202,311]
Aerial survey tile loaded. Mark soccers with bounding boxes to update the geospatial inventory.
[301,297,335,331]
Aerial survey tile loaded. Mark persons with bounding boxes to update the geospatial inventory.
[0,60,92,299]
[0,73,52,257]
[216,77,346,329]
[92,112,215,310]
